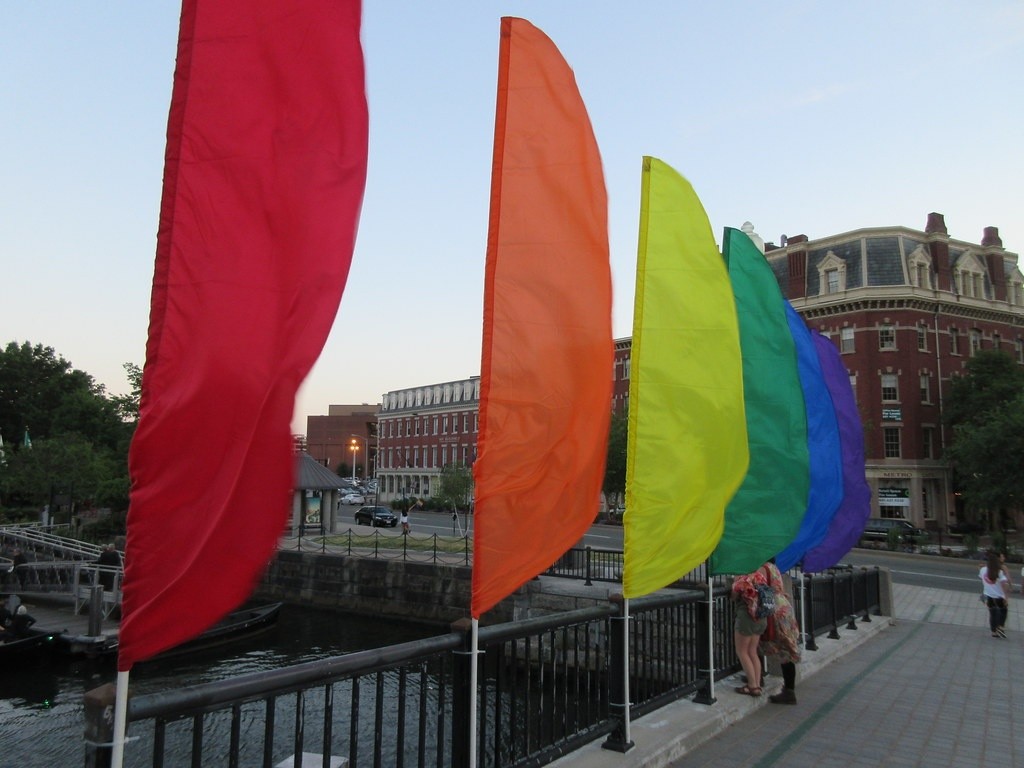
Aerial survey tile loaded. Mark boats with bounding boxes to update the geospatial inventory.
[82,601,284,677]
[0,631,64,670]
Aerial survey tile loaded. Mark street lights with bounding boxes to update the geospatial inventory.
[350,440,359,481]
[351,433,368,480]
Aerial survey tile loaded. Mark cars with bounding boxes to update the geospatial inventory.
[609,503,625,515]
[340,494,365,506]
[354,506,398,528]
[337,478,377,495]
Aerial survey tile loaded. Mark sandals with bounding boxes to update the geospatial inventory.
[735,685,763,697]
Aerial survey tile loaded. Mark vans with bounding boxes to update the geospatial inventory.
[864,517,931,544]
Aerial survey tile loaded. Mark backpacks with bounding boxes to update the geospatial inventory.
[753,582,775,619]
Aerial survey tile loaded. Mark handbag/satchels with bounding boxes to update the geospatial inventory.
[759,616,775,641]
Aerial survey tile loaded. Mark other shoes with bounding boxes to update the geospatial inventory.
[996,627,1007,638]
[402,532,406,534]
[992,635,1002,639]
[740,675,765,687]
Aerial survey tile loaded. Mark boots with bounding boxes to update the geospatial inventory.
[770,687,796,704]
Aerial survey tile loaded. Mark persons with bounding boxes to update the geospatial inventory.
[759,556,803,705]
[732,574,776,696]
[977,551,1013,640]
[400,507,409,532]
